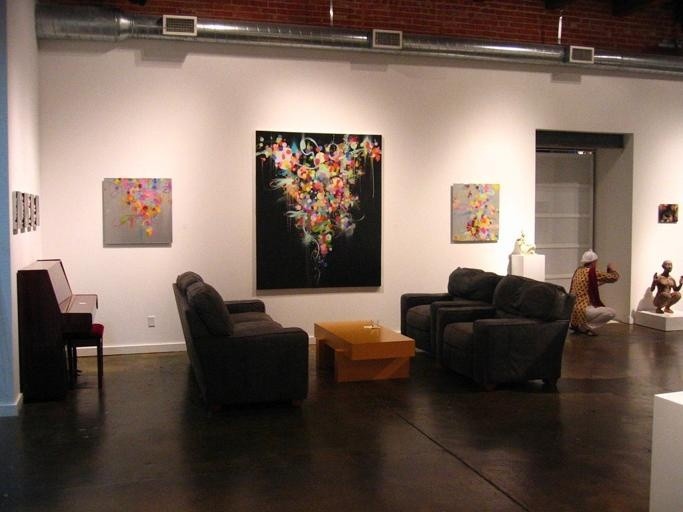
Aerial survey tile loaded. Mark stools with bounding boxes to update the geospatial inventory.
[65,324,104,383]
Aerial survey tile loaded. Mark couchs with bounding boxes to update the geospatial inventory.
[169,269,310,400]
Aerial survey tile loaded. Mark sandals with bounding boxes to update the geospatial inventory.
[569,323,601,337]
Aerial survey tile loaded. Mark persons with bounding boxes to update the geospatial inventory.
[568,250,619,336]
[650,259,683,314]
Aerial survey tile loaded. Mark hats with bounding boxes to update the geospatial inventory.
[580,249,599,264]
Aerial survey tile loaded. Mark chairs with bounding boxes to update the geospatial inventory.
[431,271,575,393]
[400,266,501,367]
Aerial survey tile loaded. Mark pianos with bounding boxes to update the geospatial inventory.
[17,259,98,400]
